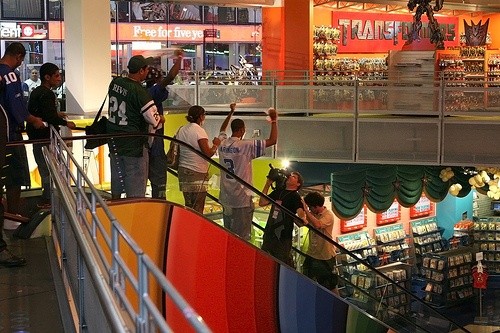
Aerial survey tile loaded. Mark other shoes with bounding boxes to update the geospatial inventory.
[0,249,28,267]
[3,215,22,230]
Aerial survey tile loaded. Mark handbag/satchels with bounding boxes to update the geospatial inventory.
[165,125,184,168]
[84,116,110,149]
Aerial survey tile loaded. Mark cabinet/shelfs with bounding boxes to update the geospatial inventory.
[340,218,500,333]
[388,50,466,116]
[462,50,500,111]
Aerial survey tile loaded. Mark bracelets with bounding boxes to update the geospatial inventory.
[271,120,276,122]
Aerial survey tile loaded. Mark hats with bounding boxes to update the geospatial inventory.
[142,67,163,84]
[126,54,153,72]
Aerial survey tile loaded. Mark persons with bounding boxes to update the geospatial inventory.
[258,171,307,270]
[106,55,165,199]
[300,191,340,296]
[218,103,278,240]
[14,70,29,101]
[25,62,75,203]
[0,182,27,267]
[0,42,45,216]
[55,69,66,99]
[24,68,41,93]
[176,105,220,215]
[142,49,183,200]
